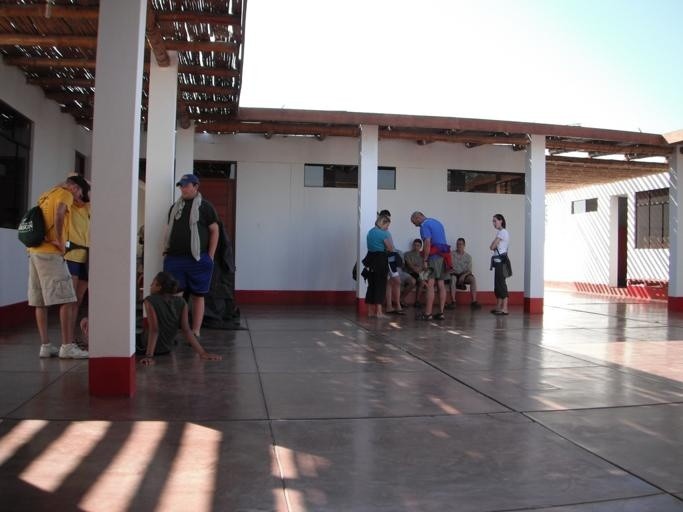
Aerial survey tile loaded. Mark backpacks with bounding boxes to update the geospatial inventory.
[17,187,61,247]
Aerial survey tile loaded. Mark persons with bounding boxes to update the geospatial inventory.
[80,271,224,368]
[352,209,509,322]
[63,171,88,351]
[161,172,220,338]
[26,175,89,361]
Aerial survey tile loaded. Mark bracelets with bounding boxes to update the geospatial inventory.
[146,351,154,358]
[163,252,168,254]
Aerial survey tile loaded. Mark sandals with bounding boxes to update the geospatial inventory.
[415,312,444,321]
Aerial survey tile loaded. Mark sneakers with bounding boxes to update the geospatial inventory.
[473,302,480,308]
[58,344,88,359]
[400,301,408,307]
[40,343,59,357]
[448,300,456,309]
[491,309,508,315]
[415,302,421,308]
[368,309,405,319]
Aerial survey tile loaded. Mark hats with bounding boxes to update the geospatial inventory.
[176,174,199,185]
[69,176,91,202]
